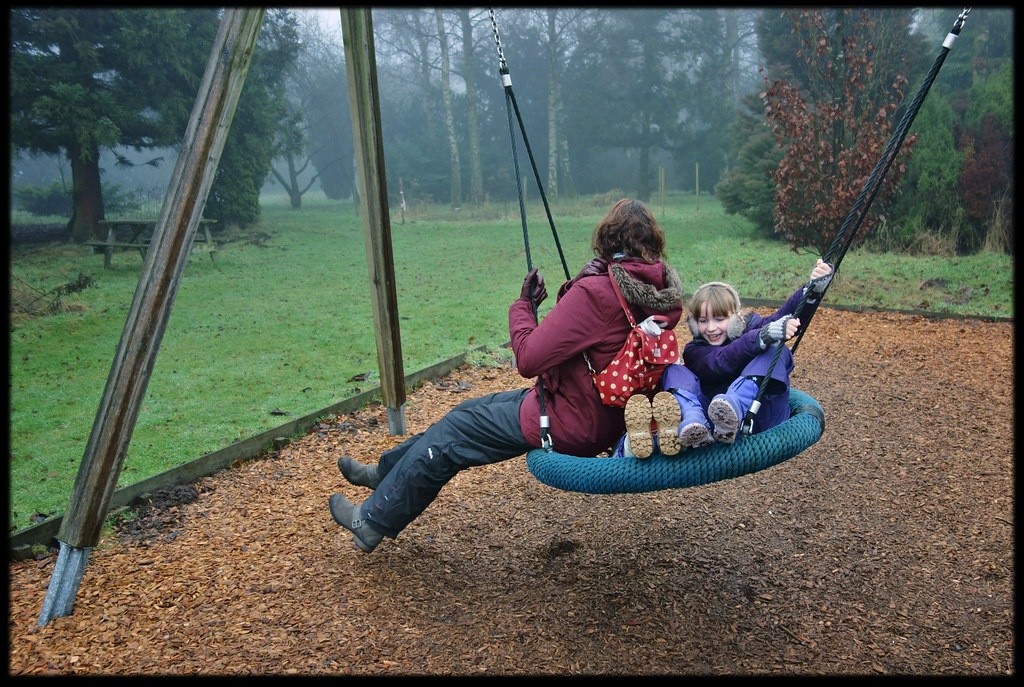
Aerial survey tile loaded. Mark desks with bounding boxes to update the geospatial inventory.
[98,219,218,270]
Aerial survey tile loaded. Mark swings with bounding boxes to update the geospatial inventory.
[487,6,974,493]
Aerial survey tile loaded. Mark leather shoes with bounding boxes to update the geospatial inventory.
[338,456,382,491]
[329,493,384,554]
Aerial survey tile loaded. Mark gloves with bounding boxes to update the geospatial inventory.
[521,267,548,308]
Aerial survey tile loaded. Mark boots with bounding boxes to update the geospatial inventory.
[708,376,759,444]
[673,389,715,448]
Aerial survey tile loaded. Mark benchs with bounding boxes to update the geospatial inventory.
[142,238,236,243]
[85,241,150,248]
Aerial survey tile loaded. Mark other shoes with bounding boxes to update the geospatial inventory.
[652,392,682,455]
[624,394,654,458]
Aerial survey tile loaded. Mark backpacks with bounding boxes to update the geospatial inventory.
[582,265,680,408]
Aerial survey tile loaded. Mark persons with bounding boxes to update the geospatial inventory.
[329,199,679,555]
[660,259,835,451]
[595,391,681,458]
